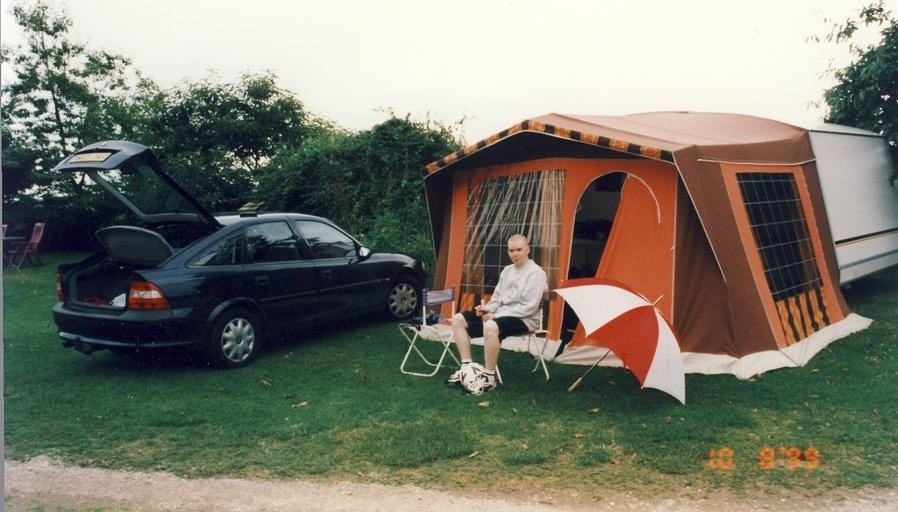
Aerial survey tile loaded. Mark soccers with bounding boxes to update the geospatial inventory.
[460,362,484,387]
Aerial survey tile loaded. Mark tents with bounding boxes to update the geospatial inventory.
[407,101,876,382]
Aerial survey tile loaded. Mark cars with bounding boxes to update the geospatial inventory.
[51,139,427,368]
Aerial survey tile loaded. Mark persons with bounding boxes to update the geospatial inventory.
[442,234,550,395]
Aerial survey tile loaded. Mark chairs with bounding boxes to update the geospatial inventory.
[398,285,558,388]
[10,221,47,270]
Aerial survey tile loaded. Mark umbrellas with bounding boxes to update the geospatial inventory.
[549,273,686,408]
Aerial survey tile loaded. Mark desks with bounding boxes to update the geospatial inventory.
[3,236,26,264]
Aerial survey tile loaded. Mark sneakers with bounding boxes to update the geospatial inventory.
[445,370,464,386]
[464,374,497,396]
